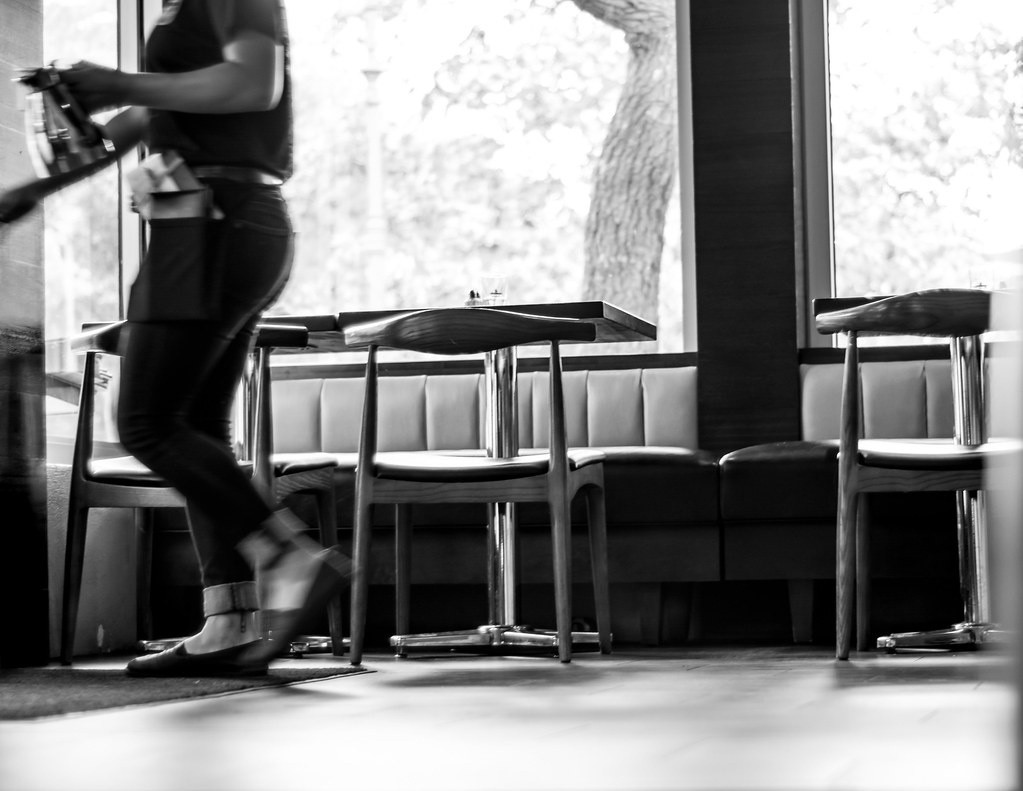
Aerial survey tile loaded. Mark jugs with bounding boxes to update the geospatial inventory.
[12,57,116,181]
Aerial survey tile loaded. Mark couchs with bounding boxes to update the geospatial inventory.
[151,356,954,652]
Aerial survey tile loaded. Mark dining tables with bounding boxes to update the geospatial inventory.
[75,313,356,665]
[340,300,656,670]
[806,294,1021,651]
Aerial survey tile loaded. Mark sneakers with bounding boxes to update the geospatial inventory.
[126,637,272,677]
[232,545,365,676]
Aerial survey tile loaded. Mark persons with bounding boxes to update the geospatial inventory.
[0,0,358,679]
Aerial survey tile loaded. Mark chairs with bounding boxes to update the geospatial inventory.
[59,319,363,674]
[814,287,1020,666]
[341,305,618,667]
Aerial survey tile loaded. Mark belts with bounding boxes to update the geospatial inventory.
[191,164,283,186]
